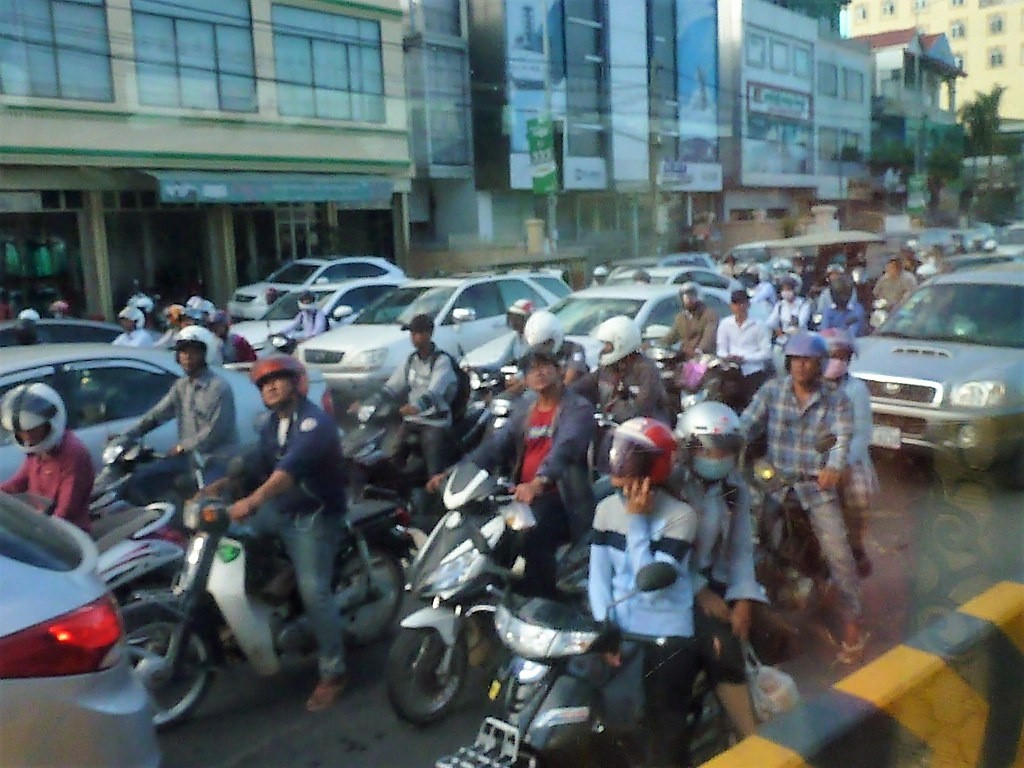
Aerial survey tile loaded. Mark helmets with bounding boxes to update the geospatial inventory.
[176,329,217,361]
[48,300,71,315]
[509,299,534,317]
[265,288,275,299]
[251,354,309,397]
[523,311,563,354]
[590,249,901,309]
[614,419,676,490]
[593,314,641,364]
[783,330,832,377]
[680,400,742,477]
[297,293,318,309]
[15,308,41,320]
[821,329,858,355]
[115,290,231,329]
[0,381,68,453]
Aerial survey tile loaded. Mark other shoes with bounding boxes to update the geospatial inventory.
[833,627,877,668]
[311,674,351,712]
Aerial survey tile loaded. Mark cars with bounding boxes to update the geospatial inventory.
[612,218,1023,265]
[297,276,564,388]
[1,313,127,346]
[0,491,161,767]
[226,278,414,360]
[0,344,273,485]
[459,281,737,375]
[227,256,406,322]
[590,266,742,306]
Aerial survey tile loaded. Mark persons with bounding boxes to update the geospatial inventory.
[2,242,1012,767]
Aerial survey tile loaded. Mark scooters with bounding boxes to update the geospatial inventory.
[585,383,640,480]
[677,345,776,416]
[340,377,491,502]
[748,435,863,661]
[92,504,188,606]
[434,521,764,768]
[89,429,247,517]
[644,338,689,430]
[455,343,522,456]
[746,259,896,357]
[386,458,622,725]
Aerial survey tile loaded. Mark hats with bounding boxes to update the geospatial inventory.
[402,314,434,333]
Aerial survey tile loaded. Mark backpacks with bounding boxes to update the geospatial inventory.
[430,349,470,423]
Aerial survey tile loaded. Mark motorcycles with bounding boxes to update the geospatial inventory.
[114,448,419,730]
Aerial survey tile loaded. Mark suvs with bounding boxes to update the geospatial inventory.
[845,259,1024,481]
